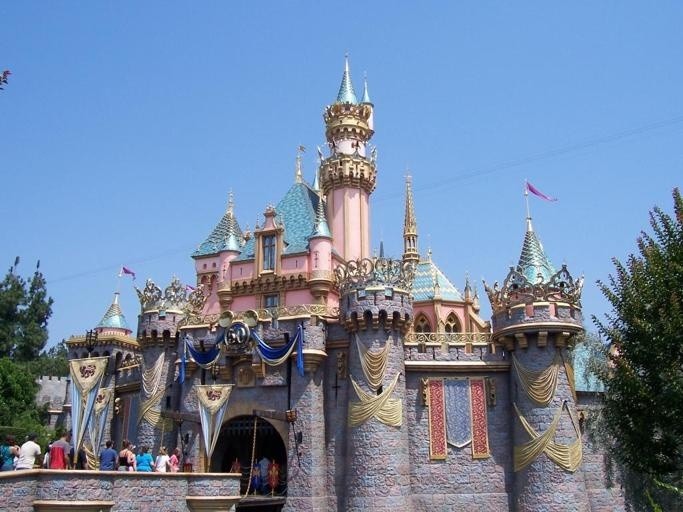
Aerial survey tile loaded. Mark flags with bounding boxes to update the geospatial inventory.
[184,285,195,292]
[528,183,556,201]
[123,267,136,281]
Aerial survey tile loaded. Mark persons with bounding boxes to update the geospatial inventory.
[0,431,89,472]
[97,440,137,472]
[152,445,171,472]
[135,444,154,472]
[167,446,183,473]
[258,452,272,496]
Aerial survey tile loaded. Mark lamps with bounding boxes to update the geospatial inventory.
[286,409,303,468]
[174,409,190,465]
